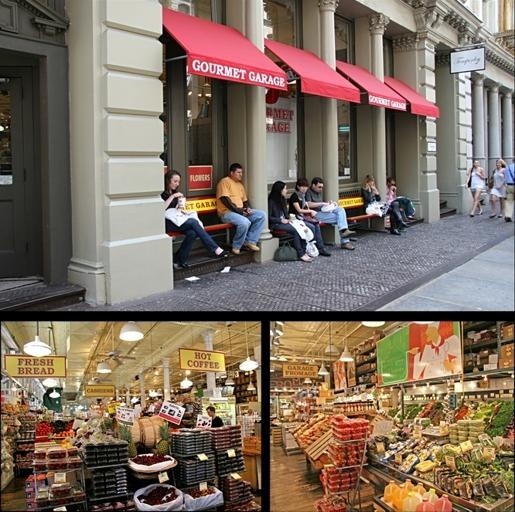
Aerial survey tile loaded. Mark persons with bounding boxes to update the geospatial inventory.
[268,180,315,263]
[488,158,508,219]
[465,158,487,217]
[305,176,357,250]
[360,173,411,235]
[205,405,224,427]
[287,177,332,256]
[386,177,417,228]
[504,156,515,224]
[216,162,266,254]
[410,320,462,380]
[160,169,229,270]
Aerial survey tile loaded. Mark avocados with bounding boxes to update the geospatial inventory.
[54,421,66,434]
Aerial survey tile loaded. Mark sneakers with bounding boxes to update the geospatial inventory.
[340,229,355,238]
[404,217,409,222]
[245,242,260,251]
[407,215,416,219]
[320,247,331,256]
[341,243,354,250]
[497,213,504,218]
[490,214,496,218]
[232,248,241,255]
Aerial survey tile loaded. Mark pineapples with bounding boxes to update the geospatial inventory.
[118,425,138,458]
[157,422,169,454]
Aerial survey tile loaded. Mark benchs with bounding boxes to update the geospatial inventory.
[337,197,378,228]
[167,194,237,253]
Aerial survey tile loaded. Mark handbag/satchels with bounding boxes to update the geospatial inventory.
[275,242,298,260]
[488,176,493,188]
[166,204,204,228]
[298,202,319,224]
[288,213,314,241]
[468,176,471,187]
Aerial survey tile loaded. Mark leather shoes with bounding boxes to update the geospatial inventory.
[391,229,400,235]
[504,217,512,222]
[402,223,409,228]
[174,263,191,271]
[213,250,229,258]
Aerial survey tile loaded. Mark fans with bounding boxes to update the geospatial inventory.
[95,322,137,366]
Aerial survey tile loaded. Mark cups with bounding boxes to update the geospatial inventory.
[177,197,186,211]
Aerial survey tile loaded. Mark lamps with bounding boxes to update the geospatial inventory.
[318,320,355,376]
[21,321,55,358]
[97,340,112,375]
[119,321,145,342]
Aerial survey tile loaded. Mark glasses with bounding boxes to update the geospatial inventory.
[319,186,323,189]
[391,184,396,186]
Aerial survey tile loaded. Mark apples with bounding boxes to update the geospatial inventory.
[36,422,55,436]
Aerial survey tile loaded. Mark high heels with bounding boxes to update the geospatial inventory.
[470,211,475,217]
[300,254,314,263]
[476,209,483,215]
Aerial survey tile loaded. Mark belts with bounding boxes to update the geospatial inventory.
[507,183,514,185]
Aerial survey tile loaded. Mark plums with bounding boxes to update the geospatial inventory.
[68,448,78,457]
[48,450,66,459]
[65,420,73,431]
[53,488,71,495]
